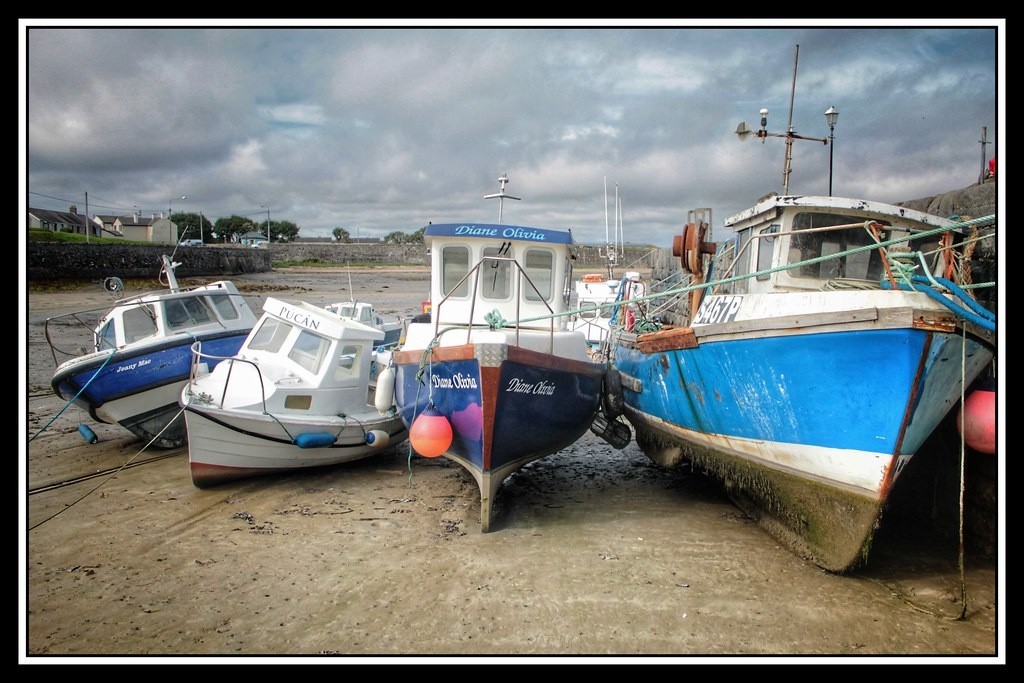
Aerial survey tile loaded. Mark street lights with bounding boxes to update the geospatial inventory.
[168,196,187,245]
[261,206,270,244]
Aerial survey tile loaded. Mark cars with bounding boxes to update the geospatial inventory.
[251,241,270,249]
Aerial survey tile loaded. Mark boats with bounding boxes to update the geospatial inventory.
[568,270,649,358]
[392,220,607,531]
[47,225,405,454]
[600,43,995,577]
[178,296,413,487]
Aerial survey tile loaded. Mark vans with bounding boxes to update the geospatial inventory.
[181,239,205,248]
